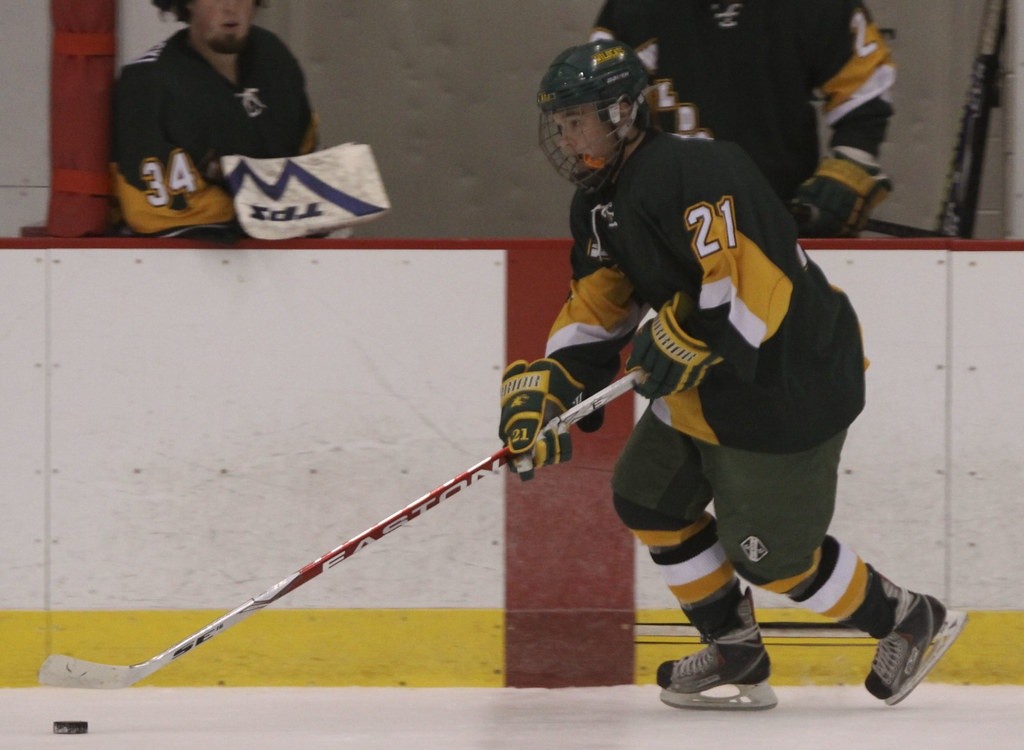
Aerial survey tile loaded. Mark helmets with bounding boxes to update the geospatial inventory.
[536,38,651,195]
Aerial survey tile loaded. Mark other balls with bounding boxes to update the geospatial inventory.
[52,719,89,735]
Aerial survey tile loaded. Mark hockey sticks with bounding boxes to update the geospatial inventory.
[36,366,647,693]
[790,201,949,238]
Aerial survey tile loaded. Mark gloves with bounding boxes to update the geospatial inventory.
[787,149,892,238]
[500,359,584,481]
[624,295,723,402]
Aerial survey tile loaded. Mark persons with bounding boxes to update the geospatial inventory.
[506,38,947,698]
[589,0,899,241]
[108,0,321,241]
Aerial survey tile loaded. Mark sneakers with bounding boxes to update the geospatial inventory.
[845,563,967,708]
[655,588,777,711]
